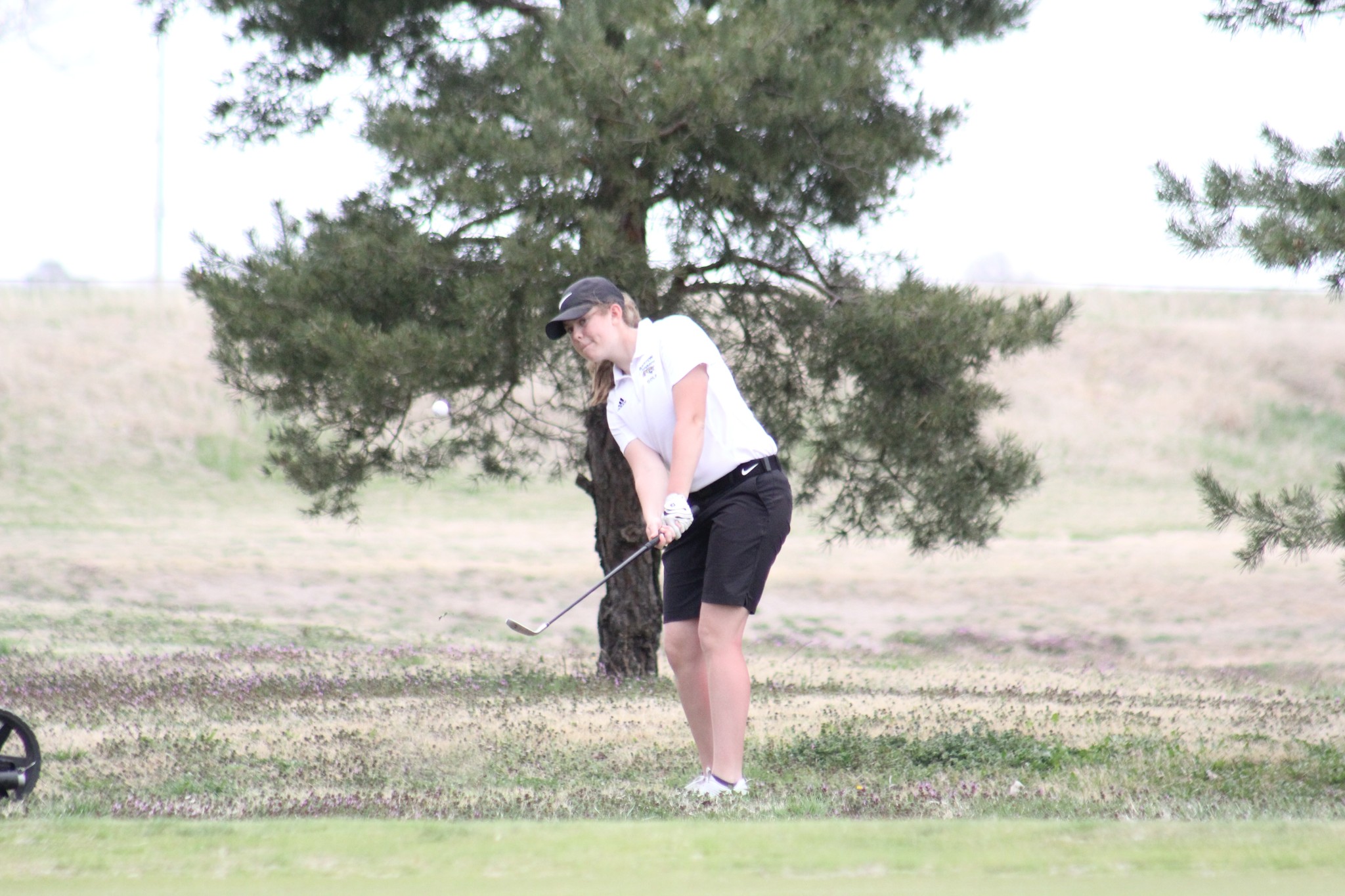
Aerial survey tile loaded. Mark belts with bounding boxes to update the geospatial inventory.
[688,455,780,507]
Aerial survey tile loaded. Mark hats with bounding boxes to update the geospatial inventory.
[545,277,624,340]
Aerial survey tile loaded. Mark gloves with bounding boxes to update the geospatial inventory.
[662,495,693,541]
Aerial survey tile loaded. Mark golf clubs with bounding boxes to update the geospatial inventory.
[506,504,701,638]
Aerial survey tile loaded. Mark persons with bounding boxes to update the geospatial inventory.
[545,276,791,803]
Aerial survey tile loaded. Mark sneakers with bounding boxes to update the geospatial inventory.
[685,777,749,798]
